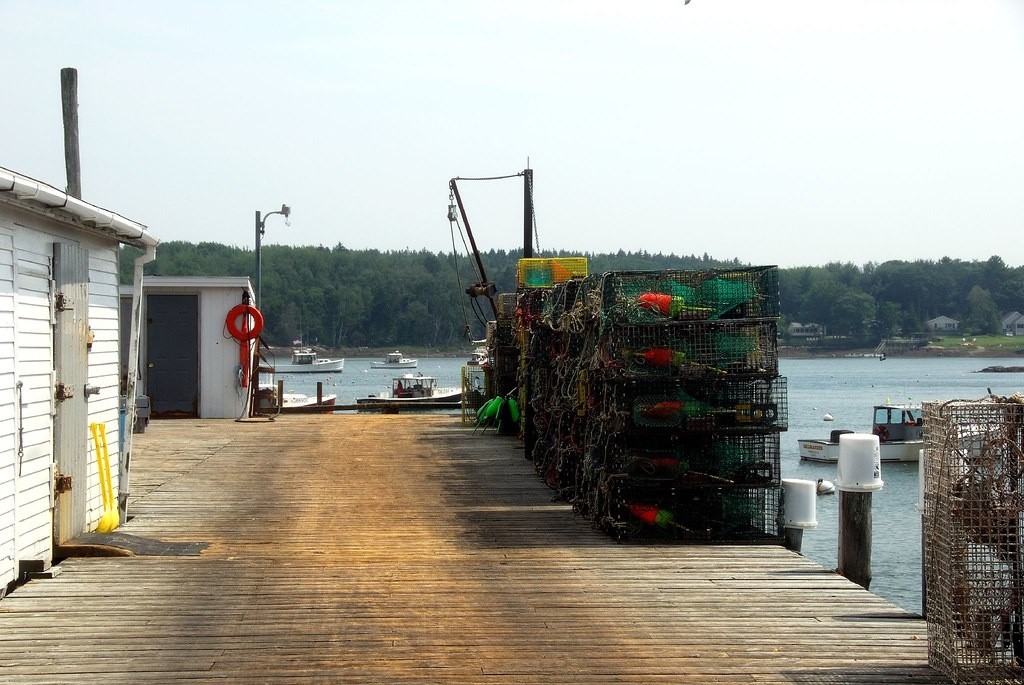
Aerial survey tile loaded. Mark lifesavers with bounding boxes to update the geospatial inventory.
[874,425,889,442]
[227,305,264,341]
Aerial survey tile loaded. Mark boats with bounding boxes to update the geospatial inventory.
[260,340,346,373]
[355,369,467,402]
[465,344,490,368]
[369,351,419,369]
[796,404,1003,463]
[842,352,881,358]
[253,384,338,415]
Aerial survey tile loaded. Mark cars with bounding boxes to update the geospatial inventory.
[959,337,975,347]
[1006,331,1014,337]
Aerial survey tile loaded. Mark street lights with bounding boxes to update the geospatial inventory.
[254,204,293,310]
